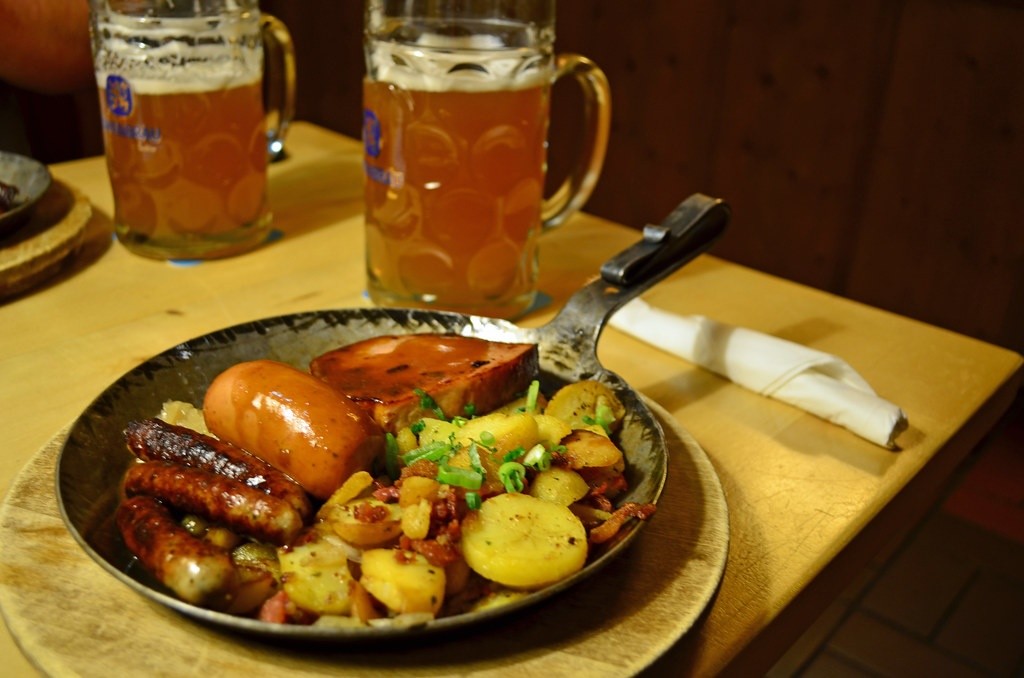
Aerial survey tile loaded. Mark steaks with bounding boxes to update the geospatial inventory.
[310,332,539,431]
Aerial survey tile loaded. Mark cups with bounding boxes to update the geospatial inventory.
[358,1,612,321]
[85,1,296,262]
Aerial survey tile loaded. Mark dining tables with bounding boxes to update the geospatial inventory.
[0,111,1024,678]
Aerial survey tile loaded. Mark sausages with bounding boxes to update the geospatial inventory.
[120,359,387,611]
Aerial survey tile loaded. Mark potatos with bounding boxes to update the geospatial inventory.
[276,382,628,618]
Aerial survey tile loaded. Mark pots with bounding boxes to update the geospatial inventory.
[54,185,732,650]
